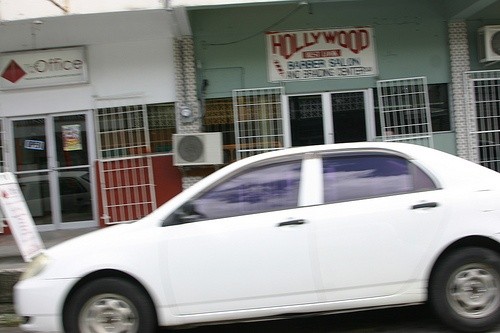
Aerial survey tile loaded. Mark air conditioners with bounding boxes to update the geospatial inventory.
[477,23,500,63]
[171,132,226,166]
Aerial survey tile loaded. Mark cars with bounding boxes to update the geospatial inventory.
[13,141,499,333]
